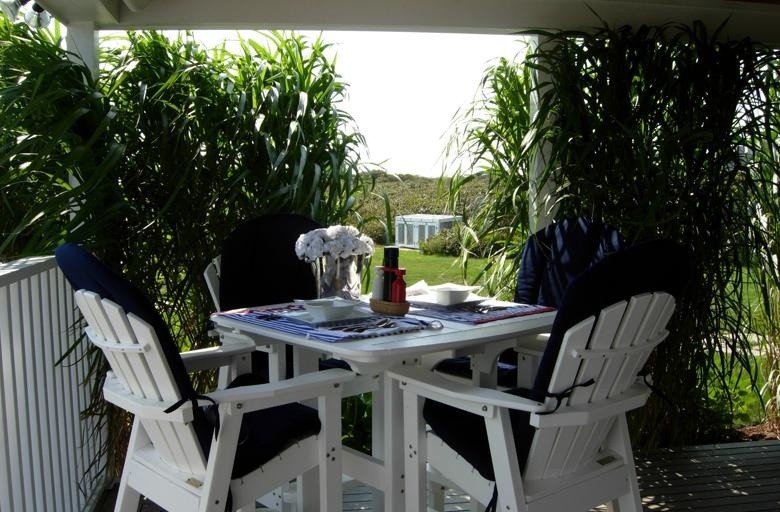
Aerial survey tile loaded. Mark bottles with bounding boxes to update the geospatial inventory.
[371,246,407,304]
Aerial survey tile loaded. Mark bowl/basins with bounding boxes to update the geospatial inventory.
[424,279,481,307]
[303,298,360,321]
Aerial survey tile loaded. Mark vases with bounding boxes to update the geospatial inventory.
[315,253,365,300]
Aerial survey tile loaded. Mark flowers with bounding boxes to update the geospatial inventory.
[295,224,374,261]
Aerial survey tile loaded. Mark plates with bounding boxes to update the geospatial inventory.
[409,295,489,314]
[283,310,374,327]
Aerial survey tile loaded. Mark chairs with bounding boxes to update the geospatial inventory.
[66,289,380,512]
[430,208,641,512]
[399,290,678,512]
[201,254,323,512]
